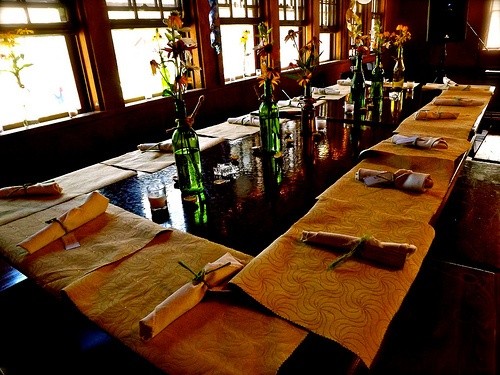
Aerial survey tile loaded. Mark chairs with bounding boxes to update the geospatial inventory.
[357,117,500,375]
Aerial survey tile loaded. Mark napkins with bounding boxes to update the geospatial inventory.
[448,85,491,91]
[15,191,107,255]
[433,97,485,107]
[227,117,260,125]
[391,134,449,150]
[337,77,352,86]
[301,229,418,269]
[277,98,302,108]
[0,182,62,198]
[355,167,433,192]
[311,86,341,95]
[138,252,245,344]
[415,109,460,119]
[137,143,174,153]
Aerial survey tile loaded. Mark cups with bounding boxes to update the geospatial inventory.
[315,116,327,131]
[407,80,414,91]
[146,181,167,211]
[345,100,354,114]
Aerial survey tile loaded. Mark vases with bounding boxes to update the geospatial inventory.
[393,47,404,92]
[350,54,367,114]
[301,79,317,131]
[371,55,383,99]
[259,79,280,152]
[173,100,205,193]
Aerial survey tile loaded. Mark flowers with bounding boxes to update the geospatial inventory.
[286,30,324,125]
[151,11,202,186]
[254,21,277,147]
[350,25,412,57]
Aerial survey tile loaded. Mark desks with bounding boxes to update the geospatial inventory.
[0,82,496,375]
